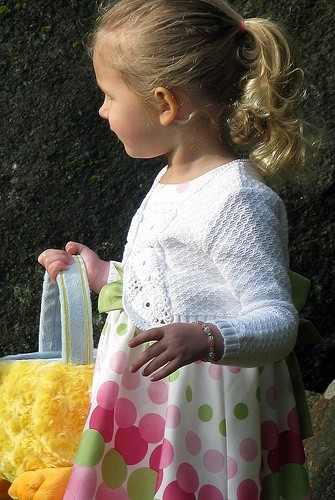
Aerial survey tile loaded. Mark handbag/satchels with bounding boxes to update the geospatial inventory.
[1,253,104,487]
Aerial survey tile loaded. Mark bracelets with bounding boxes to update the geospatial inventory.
[191,321,215,363]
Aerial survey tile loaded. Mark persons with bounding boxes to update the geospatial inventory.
[38,1,315,500]
